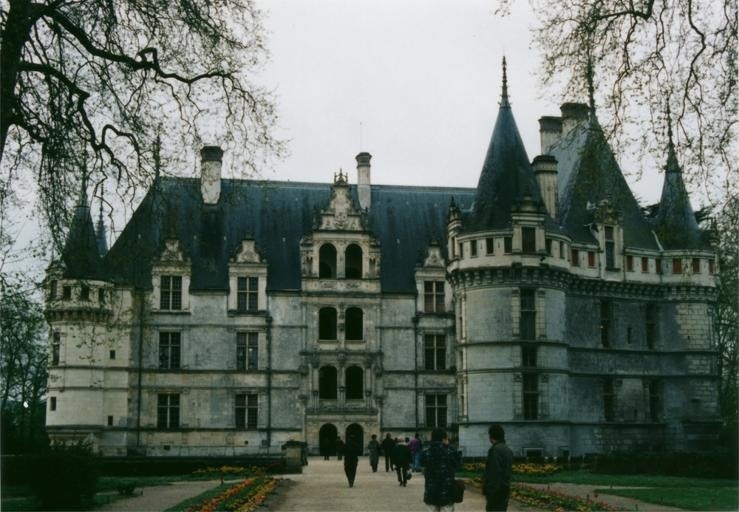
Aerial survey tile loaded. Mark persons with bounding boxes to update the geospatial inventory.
[335,436,344,460]
[368,433,422,487]
[342,434,358,487]
[420,428,464,512]
[322,436,330,460]
[481,425,513,512]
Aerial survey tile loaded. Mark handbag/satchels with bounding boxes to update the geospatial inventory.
[454,479,464,503]
